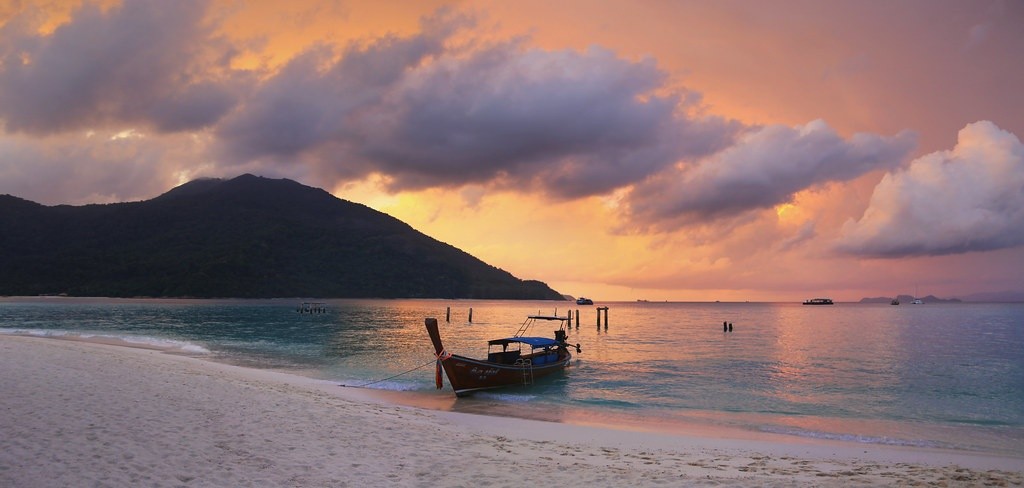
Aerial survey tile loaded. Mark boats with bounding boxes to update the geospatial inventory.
[910,299,924,304]
[576,297,593,305]
[424,315,582,398]
[803,298,833,305]
